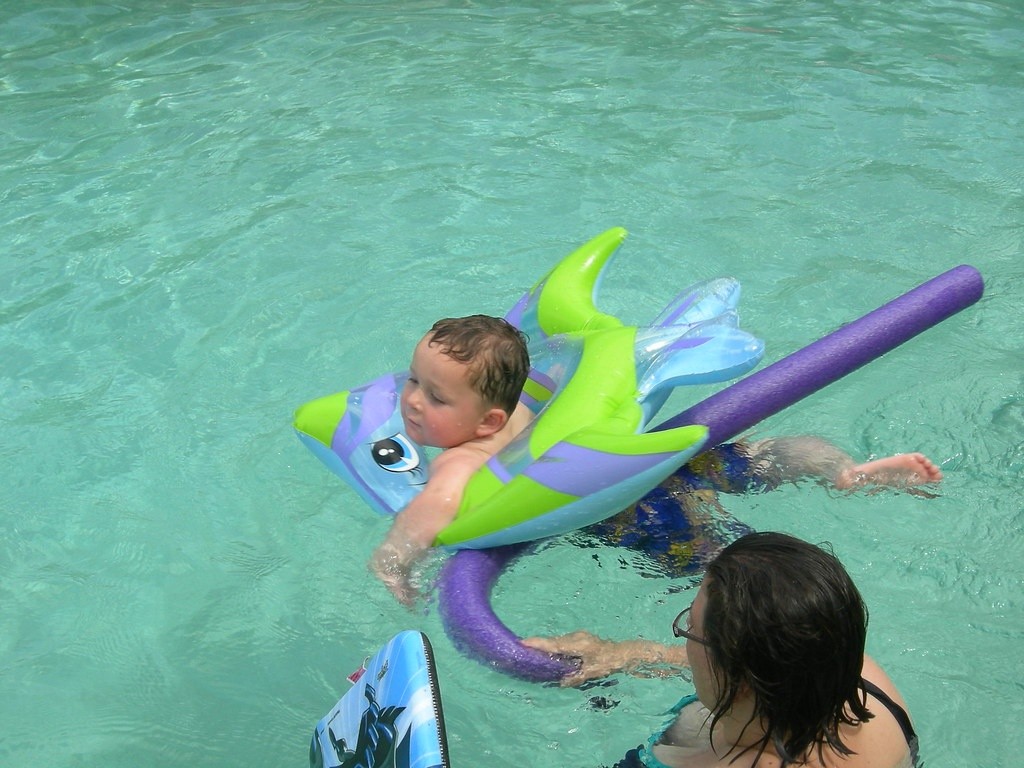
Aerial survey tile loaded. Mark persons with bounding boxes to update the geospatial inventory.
[369,314,943,549]
[518,531,925,768]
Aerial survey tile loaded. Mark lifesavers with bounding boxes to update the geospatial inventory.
[435,262,985,680]
[293,224,767,552]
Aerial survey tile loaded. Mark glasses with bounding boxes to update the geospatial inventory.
[672,607,712,648]
